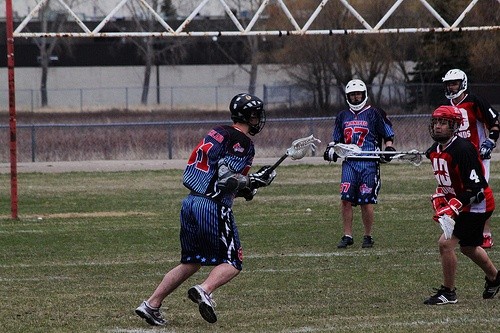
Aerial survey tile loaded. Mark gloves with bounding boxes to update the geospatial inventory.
[479,138,495,160]
[249,165,277,188]
[323,142,338,162]
[430,193,464,223]
[243,188,258,201]
[380,146,396,164]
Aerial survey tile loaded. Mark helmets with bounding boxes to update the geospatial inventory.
[431,106,463,125]
[442,69,468,99]
[345,79,368,110]
[229,93,264,123]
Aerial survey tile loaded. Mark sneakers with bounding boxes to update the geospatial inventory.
[135,300,165,326]
[483,270,500,299]
[423,284,458,305]
[188,285,217,324]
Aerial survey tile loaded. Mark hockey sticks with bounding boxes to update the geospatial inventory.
[333,143,492,159]
[268,134,322,172]
[346,149,424,166]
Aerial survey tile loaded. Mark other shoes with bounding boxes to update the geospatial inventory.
[337,235,354,249]
[481,233,494,248]
[361,235,374,248]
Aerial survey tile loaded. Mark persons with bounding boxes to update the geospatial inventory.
[441,69,500,248]
[134,93,277,326]
[323,78,396,249]
[423,106,500,305]
